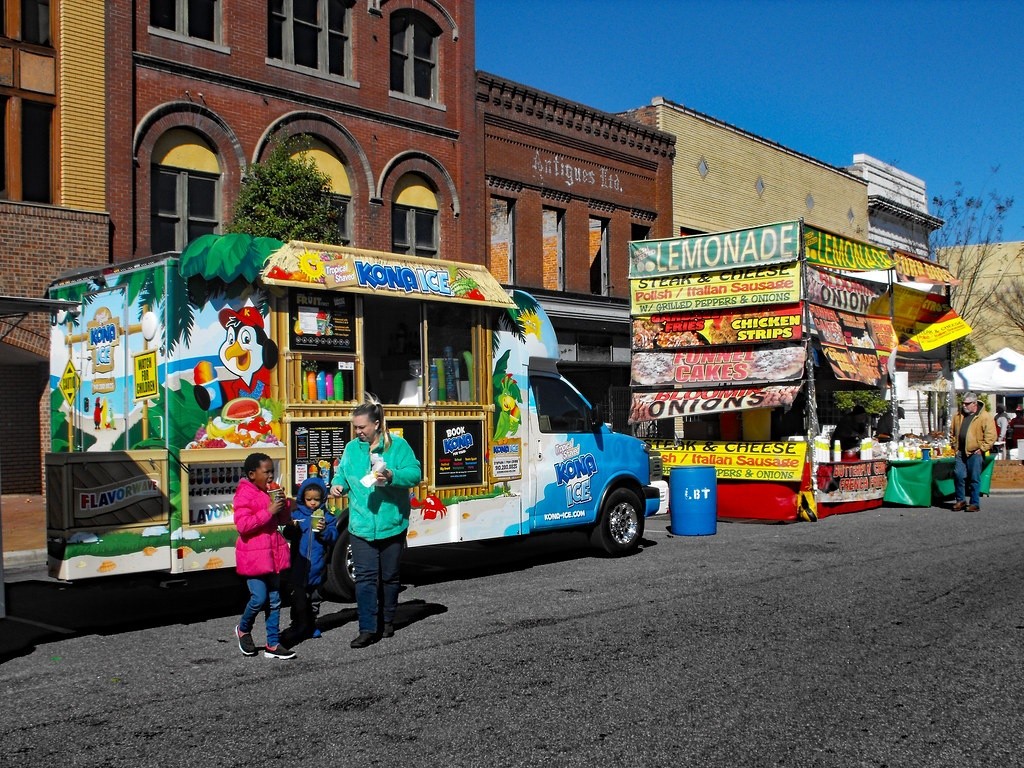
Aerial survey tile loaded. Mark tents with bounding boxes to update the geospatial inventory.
[919,347,1024,433]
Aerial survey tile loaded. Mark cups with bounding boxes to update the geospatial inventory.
[814,436,830,463]
[860,438,872,460]
[429,345,458,402]
[267,488,282,503]
[310,515,324,533]
[897,442,916,461]
[833,439,841,462]
[308,370,344,401]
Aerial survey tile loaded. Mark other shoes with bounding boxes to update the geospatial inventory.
[304,626,321,637]
[288,628,304,638]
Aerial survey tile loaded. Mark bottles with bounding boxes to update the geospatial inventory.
[917,437,955,460]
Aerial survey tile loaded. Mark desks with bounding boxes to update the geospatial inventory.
[883,455,994,507]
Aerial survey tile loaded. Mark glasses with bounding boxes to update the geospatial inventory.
[961,402,972,406]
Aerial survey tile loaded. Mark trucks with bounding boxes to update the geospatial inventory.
[42,236,670,605]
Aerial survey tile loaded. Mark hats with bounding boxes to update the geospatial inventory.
[898,407,904,419]
[853,405,866,416]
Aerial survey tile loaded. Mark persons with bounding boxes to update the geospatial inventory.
[283,478,339,637]
[949,392,997,513]
[233,453,296,659]
[876,407,905,437]
[330,403,421,647]
[831,405,866,451]
[1008,406,1024,448]
[994,403,1010,460]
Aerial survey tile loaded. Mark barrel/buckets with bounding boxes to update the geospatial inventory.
[669,465,717,536]
[719,407,775,442]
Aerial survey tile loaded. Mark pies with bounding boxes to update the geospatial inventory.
[749,344,804,379]
[632,352,676,385]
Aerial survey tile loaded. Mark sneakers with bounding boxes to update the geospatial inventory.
[952,501,965,511]
[966,504,980,511]
[350,632,378,647]
[264,643,296,659]
[377,623,394,637]
[236,625,255,655]
